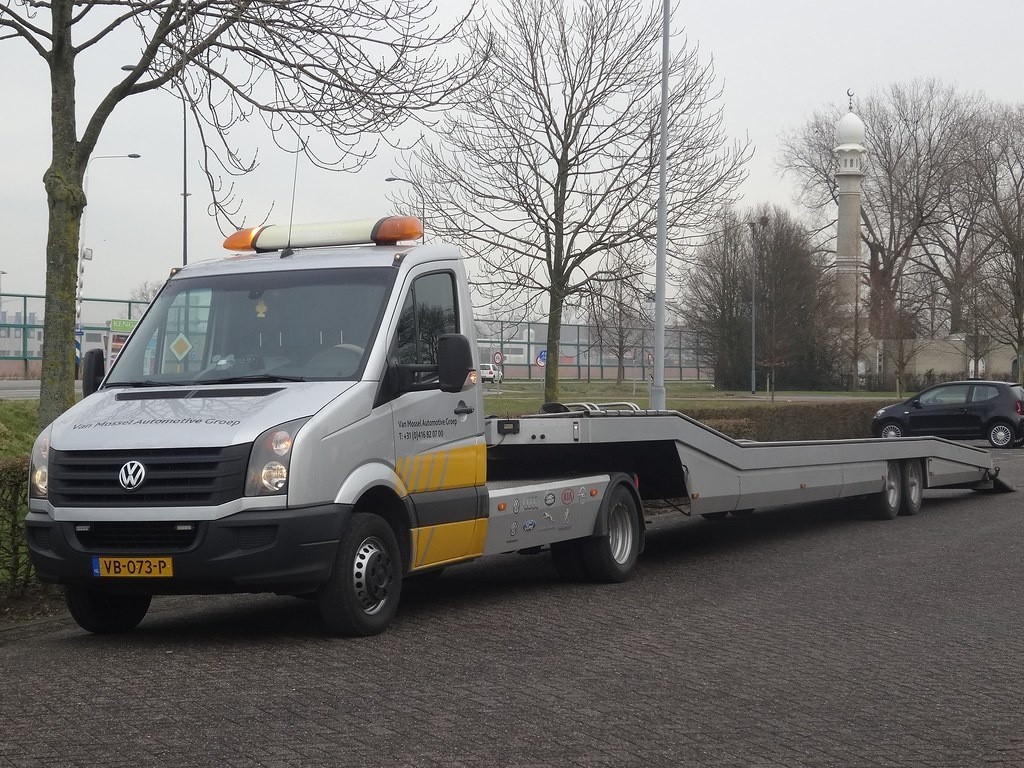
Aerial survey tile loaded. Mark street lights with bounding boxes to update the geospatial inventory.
[122,65,188,374]
[749,222,756,394]
[76,154,142,343]
[386,178,425,244]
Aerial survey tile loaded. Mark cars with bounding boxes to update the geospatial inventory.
[871,381,1024,448]
[480,364,503,384]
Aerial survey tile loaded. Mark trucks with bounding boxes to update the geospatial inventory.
[26,216,1016,638]
